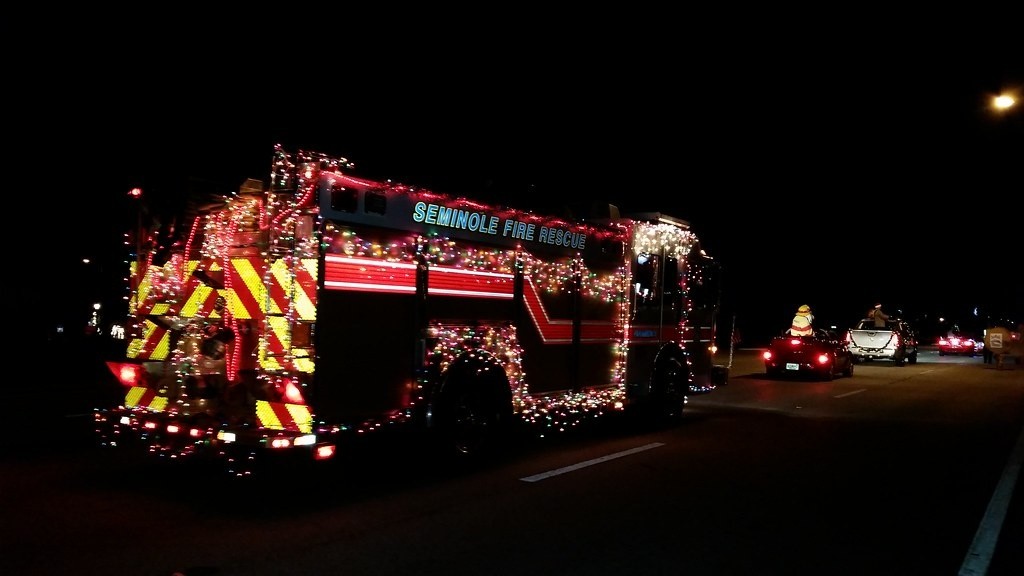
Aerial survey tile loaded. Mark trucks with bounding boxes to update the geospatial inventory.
[96,145,736,480]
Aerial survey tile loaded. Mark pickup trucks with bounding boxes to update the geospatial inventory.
[846,317,918,366]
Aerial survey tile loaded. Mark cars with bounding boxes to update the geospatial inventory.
[763,326,855,380]
[982,327,1024,364]
[938,334,976,358]
